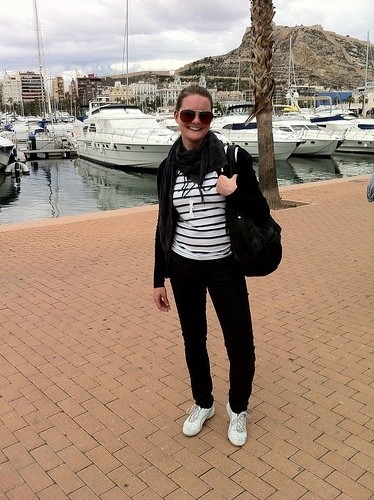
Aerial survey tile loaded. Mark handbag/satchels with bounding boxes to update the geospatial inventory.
[224,145,282,277]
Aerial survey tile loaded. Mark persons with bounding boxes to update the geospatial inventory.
[152,84,271,446]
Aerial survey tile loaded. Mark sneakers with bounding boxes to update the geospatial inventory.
[226,401,251,446]
[182,402,215,437]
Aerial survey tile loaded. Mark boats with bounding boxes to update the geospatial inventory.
[0,0,374,167]
[75,104,234,169]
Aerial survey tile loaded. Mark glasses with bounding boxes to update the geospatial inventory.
[177,109,214,125]
